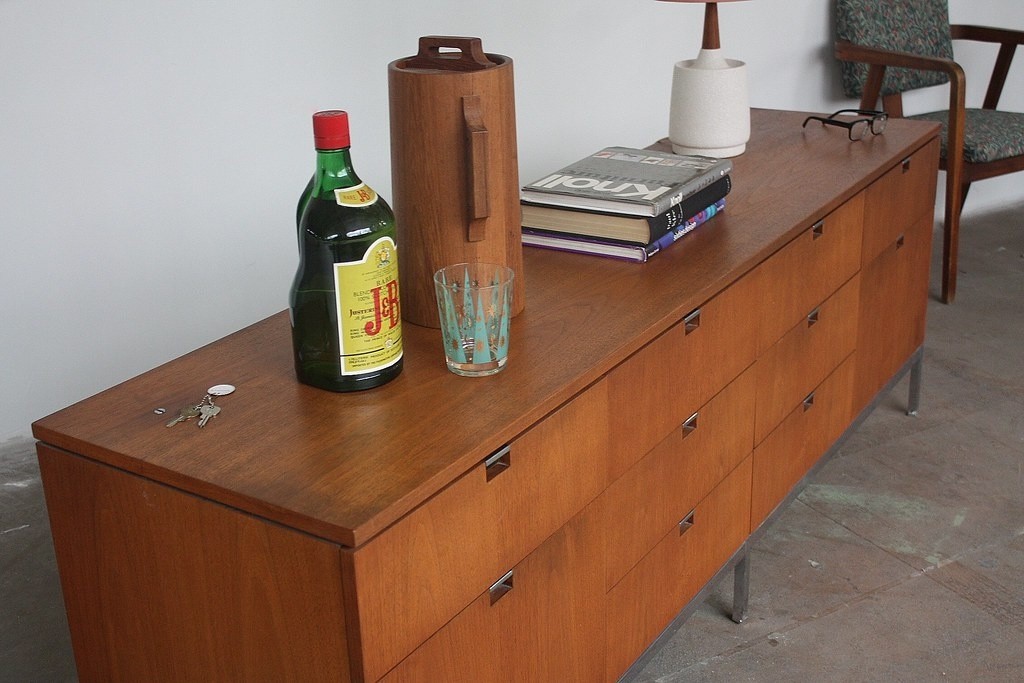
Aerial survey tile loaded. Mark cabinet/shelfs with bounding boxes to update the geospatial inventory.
[30,109,943,683]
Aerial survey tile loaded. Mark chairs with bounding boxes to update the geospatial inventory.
[828,0,1024,304]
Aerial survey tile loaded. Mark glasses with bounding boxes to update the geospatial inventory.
[803,110,889,141]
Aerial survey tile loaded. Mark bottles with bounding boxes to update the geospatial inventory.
[289,112,404,392]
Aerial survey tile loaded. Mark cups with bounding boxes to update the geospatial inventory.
[434,261,514,378]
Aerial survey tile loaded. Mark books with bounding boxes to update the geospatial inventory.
[520,147,732,262]
